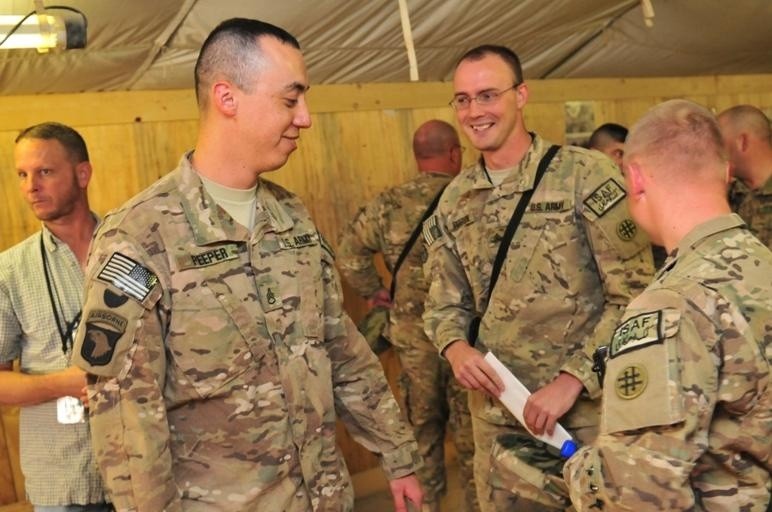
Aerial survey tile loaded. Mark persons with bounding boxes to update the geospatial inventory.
[582,123,630,177]
[419,44,656,512]
[0,121,118,512]
[577,96,771,512]
[713,103,771,253]
[333,117,485,510]
[68,14,426,512]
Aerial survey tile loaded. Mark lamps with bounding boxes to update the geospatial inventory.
[1,1,87,54]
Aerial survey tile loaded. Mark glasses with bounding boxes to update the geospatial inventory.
[447,83,515,111]
